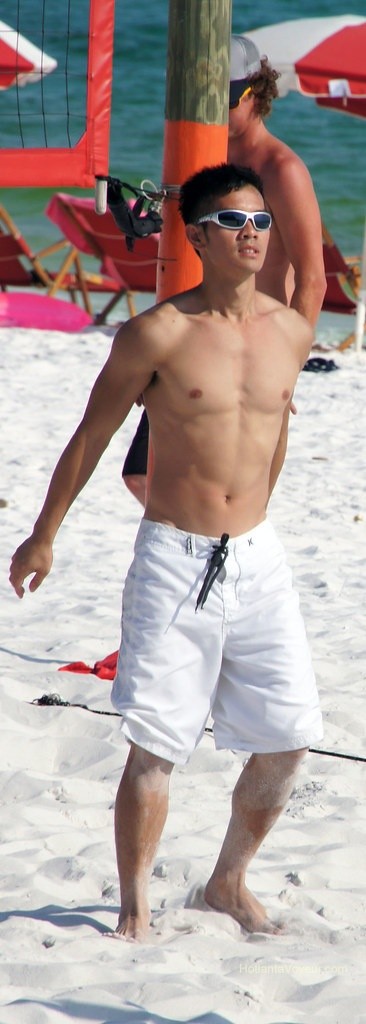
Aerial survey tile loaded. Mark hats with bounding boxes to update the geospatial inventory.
[230,33,261,103]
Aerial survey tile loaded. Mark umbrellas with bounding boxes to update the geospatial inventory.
[0,19,58,90]
[241,13,366,118]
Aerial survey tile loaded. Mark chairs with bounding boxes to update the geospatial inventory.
[47,190,165,292]
[318,220,362,310]
[0,203,137,323]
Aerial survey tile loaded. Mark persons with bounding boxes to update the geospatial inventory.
[8,165,324,944]
[93,33,330,682]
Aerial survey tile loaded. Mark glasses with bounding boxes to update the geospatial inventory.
[192,209,272,231]
[229,85,252,114]
